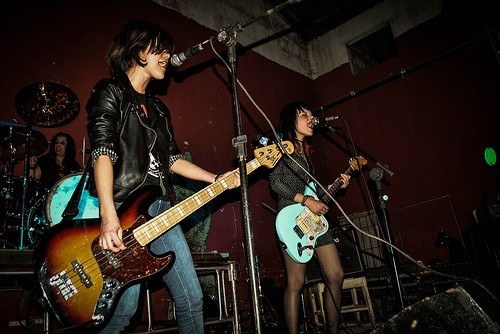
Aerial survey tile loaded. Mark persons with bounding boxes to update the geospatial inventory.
[84,18,241,334]
[29,132,81,190]
[268,101,351,334]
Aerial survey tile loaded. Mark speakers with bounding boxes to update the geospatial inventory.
[304,224,367,285]
[371,268,499,334]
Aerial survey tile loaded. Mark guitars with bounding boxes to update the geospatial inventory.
[274,156,370,264]
[33,136,295,334]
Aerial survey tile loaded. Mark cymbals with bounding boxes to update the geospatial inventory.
[0,125,48,160]
[14,82,80,128]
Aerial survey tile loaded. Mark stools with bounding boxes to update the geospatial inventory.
[307,274,378,331]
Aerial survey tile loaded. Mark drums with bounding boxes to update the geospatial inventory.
[0,176,43,233]
[26,172,99,245]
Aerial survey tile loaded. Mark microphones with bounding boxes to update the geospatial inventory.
[311,116,339,126]
[170,44,206,67]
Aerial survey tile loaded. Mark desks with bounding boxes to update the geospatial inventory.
[42,258,242,334]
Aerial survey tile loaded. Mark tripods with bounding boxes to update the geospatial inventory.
[0,101,49,250]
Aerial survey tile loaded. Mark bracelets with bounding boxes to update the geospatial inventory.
[301,195,314,206]
[29,166,36,170]
[215,173,224,182]
